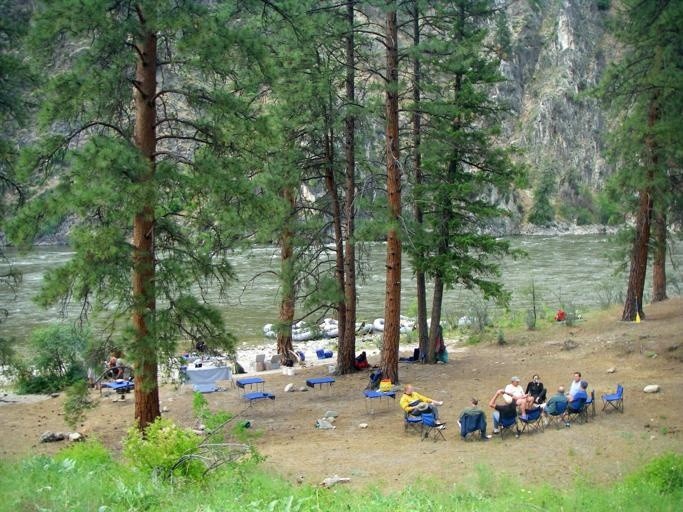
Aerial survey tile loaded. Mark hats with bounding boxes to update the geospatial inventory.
[580,379,588,389]
[511,376,521,382]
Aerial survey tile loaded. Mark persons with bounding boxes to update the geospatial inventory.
[400,384,447,430]
[196,336,205,361]
[457,396,487,430]
[489,372,589,434]
[110,357,131,380]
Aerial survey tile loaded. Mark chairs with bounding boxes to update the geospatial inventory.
[405,384,624,443]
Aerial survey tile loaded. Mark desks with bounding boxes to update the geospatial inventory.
[185,364,232,388]
[236,378,275,406]
[365,390,395,414]
[306,378,335,392]
